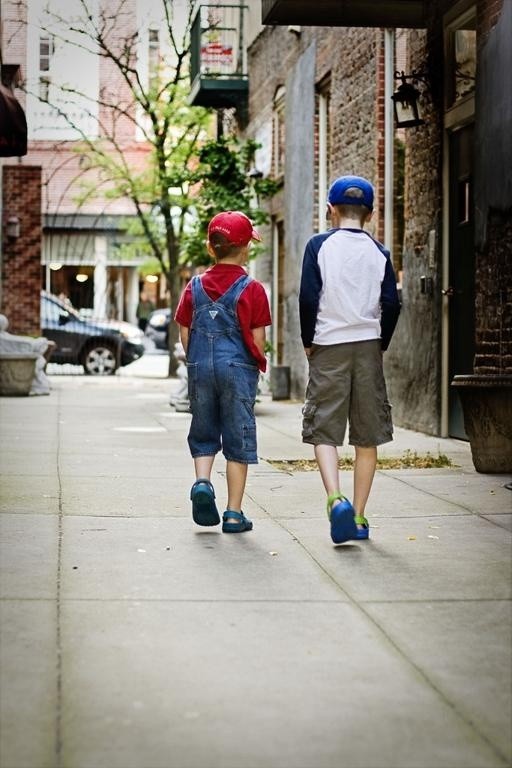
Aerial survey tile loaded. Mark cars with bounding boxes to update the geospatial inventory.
[39,289,173,376]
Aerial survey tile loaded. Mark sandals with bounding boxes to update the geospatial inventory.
[222,507,253,534]
[351,515,371,541]
[188,478,221,528]
[325,493,357,545]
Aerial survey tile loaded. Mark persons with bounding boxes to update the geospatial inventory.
[173,210,272,532]
[299,176,401,543]
[52,282,80,311]
[135,284,171,332]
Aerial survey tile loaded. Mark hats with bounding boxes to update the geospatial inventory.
[207,210,263,245]
[325,174,375,221]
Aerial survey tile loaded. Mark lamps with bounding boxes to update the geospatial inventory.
[389,43,443,132]
[3,214,21,255]
